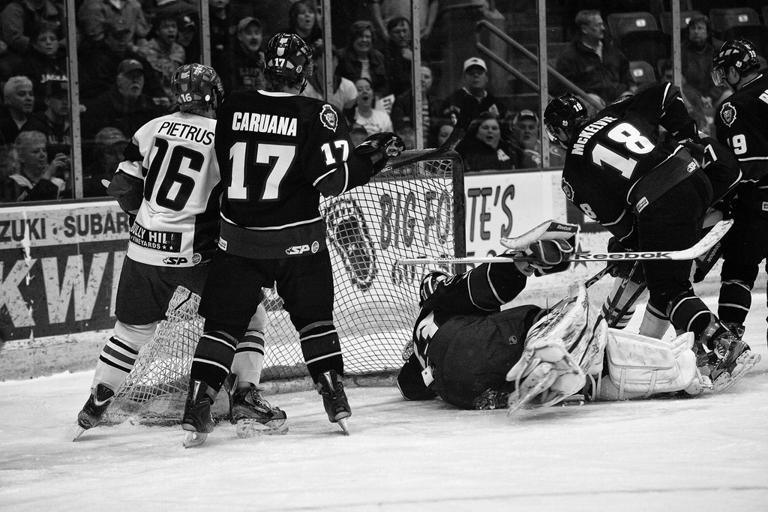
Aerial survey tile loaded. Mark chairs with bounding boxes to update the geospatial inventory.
[548,0,768,124]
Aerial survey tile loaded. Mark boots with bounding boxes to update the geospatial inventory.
[677,320,750,383]
[507,362,558,408]
[182,379,215,433]
[78,384,114,429]
[319,369,351,422]
[224,374,286,424]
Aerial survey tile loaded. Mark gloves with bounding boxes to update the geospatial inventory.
[511,239,573,276]
[355,132,406,171]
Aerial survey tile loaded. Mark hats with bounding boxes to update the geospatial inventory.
[40,71,68,95]
[464,57,487,72]
[238,17,262,33]
[95,126,130,145]
[513,109,539,128]
[118,59,143,75]
[103,14,130,33]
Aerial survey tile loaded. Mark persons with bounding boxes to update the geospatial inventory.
[702,37,768,366]
[544,83,761,382]
[181,32,405,432]
[396,219,712,411]
[592,118,744,340]
[70,63,288,430]
[0,0,766,205]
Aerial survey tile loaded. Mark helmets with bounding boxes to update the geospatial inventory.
[544,96,588,151]
[171,63,225,112]
[712,38,760,88]
[265,33,314,92]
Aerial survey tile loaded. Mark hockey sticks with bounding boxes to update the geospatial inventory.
[375,94,479,176]
[397,217,735,265]
